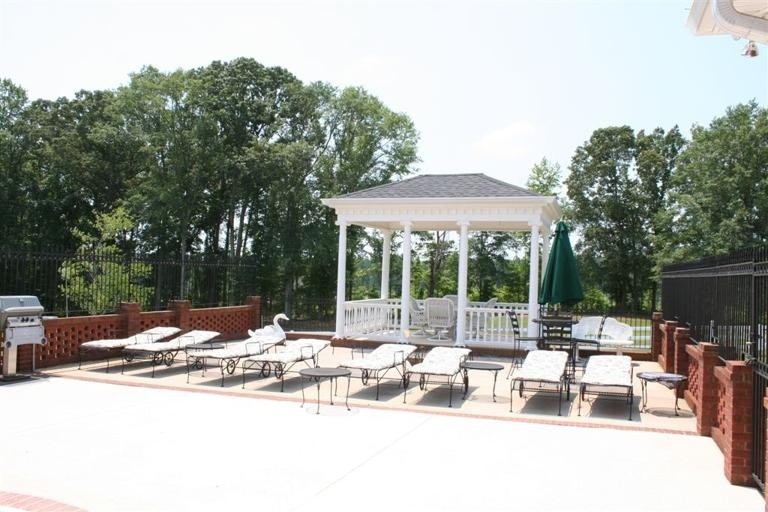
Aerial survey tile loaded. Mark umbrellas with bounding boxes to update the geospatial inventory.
[538,222,585,351]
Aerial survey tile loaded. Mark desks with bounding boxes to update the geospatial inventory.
[184,343,225,378]
[460,362,504,402]
[298,368,352,414]
[636,372,688,416]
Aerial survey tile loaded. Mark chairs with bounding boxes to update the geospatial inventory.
[186,335,286,387]
[403,346,472,407]
[408,294,498,341]
[121,329,222,378]
[335,343,418,400]
[507,311,634,420]
[242,338,332,392]
[78,326,182,373]
